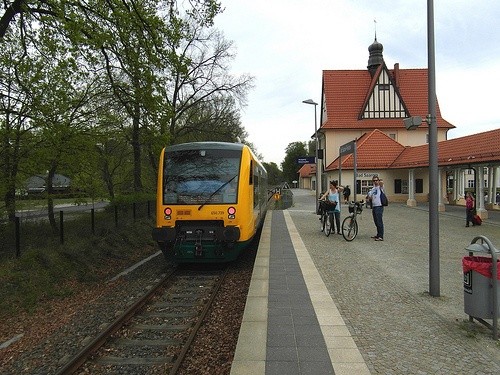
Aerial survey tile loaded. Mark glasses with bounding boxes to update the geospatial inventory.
[373,180,378,182]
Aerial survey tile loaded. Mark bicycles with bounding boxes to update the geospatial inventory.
[318,200,338,237]
[342,199,365,242]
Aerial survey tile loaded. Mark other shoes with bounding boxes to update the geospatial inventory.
[472,222,475,226]
[465,224,470,227]
[337,232,342,235]
[331,231,335,234]
[371,235,384,241]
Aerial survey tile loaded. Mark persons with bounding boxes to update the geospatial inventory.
[464,191,477,227]
[484,194,489,203]
[496,192,500,206]
[342,184,351,203]
[317,179,344,235]
[366,174,385,241]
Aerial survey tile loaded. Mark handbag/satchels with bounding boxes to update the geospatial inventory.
[343,191,347,195]
[379,186,388,206]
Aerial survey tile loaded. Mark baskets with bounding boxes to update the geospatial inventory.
[351,204,362,214]
[324,203,336,211]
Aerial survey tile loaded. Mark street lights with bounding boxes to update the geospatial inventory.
[302,99,319,215]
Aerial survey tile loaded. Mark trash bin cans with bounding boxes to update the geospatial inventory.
[462,235,499,339]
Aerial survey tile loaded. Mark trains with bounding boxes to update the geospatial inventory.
[153,141,268,269]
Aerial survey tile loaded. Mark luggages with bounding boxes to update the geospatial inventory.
[471,214,482,225]
